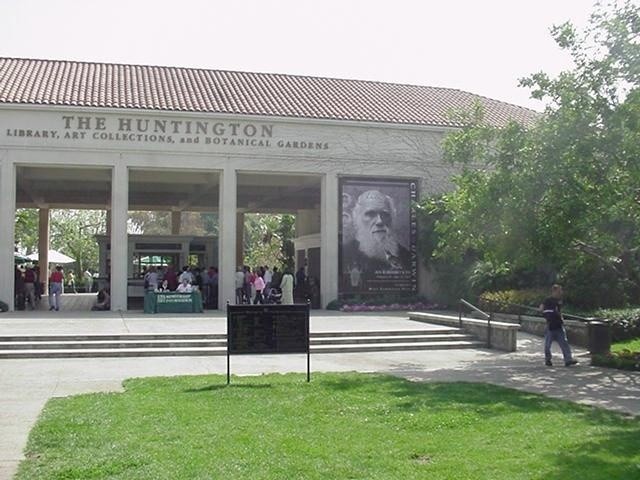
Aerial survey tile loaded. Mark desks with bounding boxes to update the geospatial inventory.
[141,291,205,313]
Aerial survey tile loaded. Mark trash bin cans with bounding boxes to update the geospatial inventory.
[583,317,611,355]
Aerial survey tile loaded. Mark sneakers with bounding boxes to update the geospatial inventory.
[565,360,577,365]
[546,360,553,366]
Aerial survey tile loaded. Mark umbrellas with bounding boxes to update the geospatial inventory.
[26,249,77,263]
[14,251,32,264]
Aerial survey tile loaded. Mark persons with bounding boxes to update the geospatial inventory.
[539,285,578,366]
[343,189,412,292]
[139,263,305,309]
[15,263,110,311]
